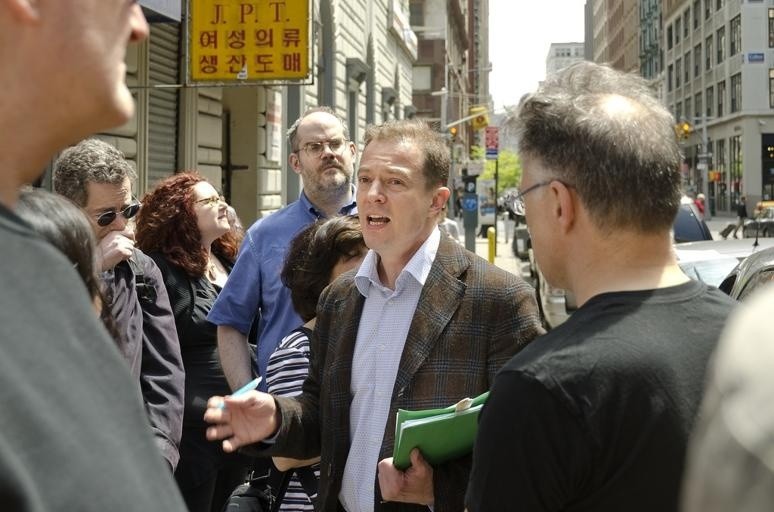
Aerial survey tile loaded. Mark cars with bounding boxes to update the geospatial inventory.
[668,197,774,289]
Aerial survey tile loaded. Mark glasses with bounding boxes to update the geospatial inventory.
[92,193,142,228]
[190,194,226,207]
[506,179,572,217]
[294,137,346,159]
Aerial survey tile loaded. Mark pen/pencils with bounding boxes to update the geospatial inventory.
[220,376,262,411]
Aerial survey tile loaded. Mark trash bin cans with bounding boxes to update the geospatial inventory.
[480,224,494,238]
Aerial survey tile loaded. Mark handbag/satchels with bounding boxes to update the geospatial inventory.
[220,474,277,512]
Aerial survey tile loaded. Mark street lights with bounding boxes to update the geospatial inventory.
[441,111,493,217]
